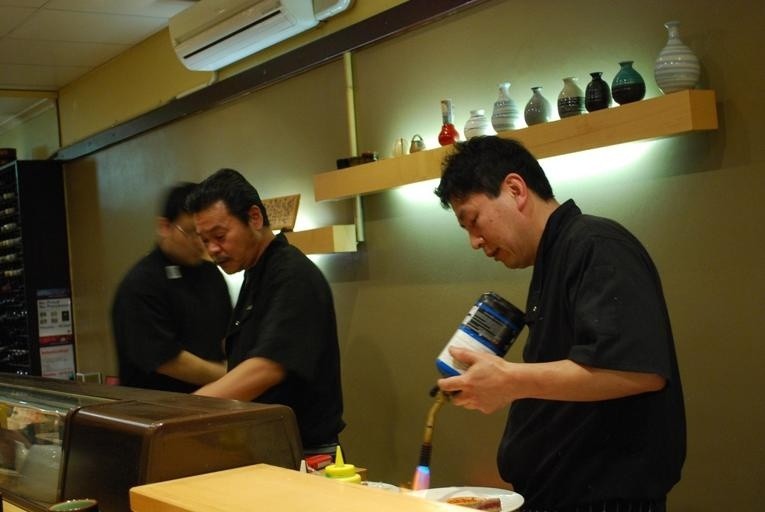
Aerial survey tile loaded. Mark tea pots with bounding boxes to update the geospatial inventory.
[12,440,63,504]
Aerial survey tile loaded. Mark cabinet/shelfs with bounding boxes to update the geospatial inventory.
[2,160,76,376]
[284,88,721,254]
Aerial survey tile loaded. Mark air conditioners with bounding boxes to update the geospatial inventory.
[167,0,321,73]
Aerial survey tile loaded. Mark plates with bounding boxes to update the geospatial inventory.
[403,483,526,512]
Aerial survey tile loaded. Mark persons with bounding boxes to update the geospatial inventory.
[184,168,347,464]
[112,182,232,393]
[433,135,686,512]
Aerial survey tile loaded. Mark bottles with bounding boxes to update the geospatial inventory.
[436,19,703,146]
